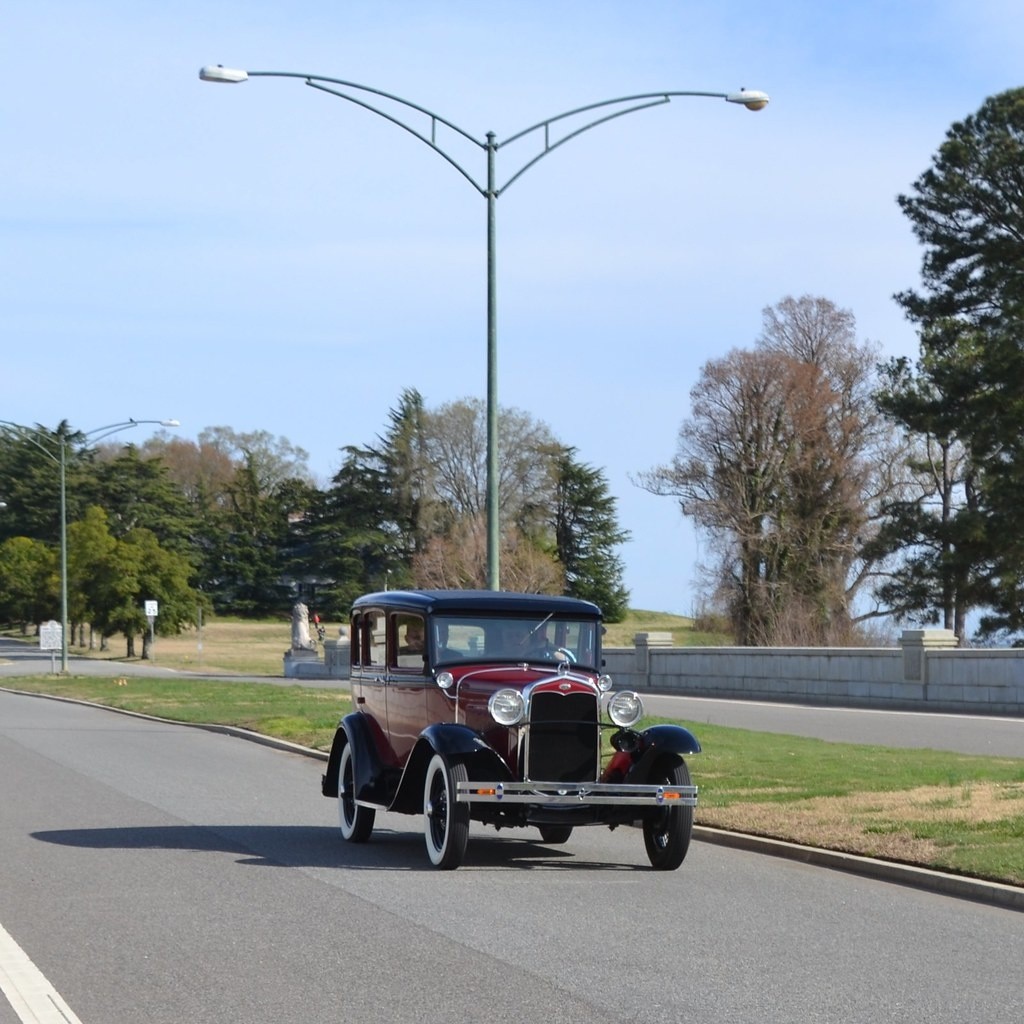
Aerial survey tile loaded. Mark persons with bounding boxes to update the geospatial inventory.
[520,621,571,662]
[399,616,425,655]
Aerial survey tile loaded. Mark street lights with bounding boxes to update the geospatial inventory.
[202,61,769,592]
[0,417,179,670]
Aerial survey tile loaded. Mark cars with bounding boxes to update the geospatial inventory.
[323,590,702,871]
